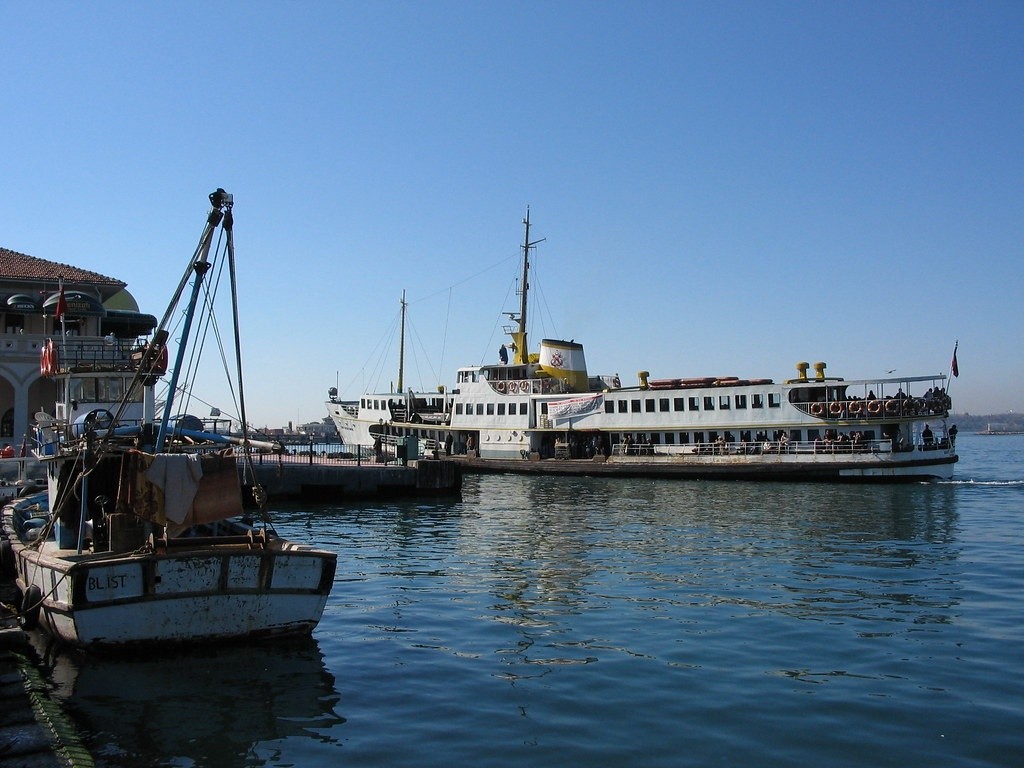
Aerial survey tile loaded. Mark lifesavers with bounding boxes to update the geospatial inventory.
[496,379,529,392]
[19,584,42,632]
[139,342,169,387]
[39,340,58,378]
[810,400,923,416]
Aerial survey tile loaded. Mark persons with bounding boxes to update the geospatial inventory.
[445,387,958,459]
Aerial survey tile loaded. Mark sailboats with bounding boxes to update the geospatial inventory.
[1,189,338,664]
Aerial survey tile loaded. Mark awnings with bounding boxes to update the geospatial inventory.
[7,291,156,336]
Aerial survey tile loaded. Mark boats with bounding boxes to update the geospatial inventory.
[324,203,960,483]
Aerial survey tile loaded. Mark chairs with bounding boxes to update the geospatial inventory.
[35,412,69,457]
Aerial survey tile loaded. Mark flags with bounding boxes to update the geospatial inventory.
[56,277,68,321]
[952,343,959,378]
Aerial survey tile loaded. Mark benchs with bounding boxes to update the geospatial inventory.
[790,444,813,452]
